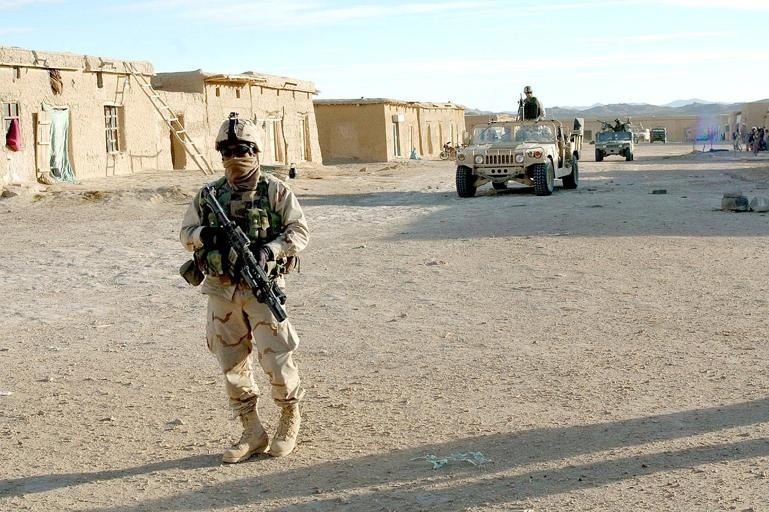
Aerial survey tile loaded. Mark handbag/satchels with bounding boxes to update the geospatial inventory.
[179,260,204,286]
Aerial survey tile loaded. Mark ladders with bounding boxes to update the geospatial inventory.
[124,61,214,175]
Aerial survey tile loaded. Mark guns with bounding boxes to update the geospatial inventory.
[596,120,611,130]
[195,186,287,322]
[517,93,526,107]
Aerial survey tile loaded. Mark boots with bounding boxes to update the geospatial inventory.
[221,402,301,463]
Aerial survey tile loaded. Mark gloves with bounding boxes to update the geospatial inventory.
[257,248,270,271]
[200,226,228,247]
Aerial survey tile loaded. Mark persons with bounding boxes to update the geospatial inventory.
[486,129,497,141]
[541,126,552,138]
[517,85,546,123]
[178,118,311,464]
[614,118,625,132]
[733,126,769,154]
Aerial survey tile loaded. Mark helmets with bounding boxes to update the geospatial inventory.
[524,86,534,93]
[215,118,261,152]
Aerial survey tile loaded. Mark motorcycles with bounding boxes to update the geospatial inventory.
[745,128,768,152]
[440,142,460,160]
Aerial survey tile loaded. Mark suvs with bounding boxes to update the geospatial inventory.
[594,131,635,161]
[456,115,579,197]
[649,128,667,143]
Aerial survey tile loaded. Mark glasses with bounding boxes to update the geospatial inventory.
[223,148,254,157]
